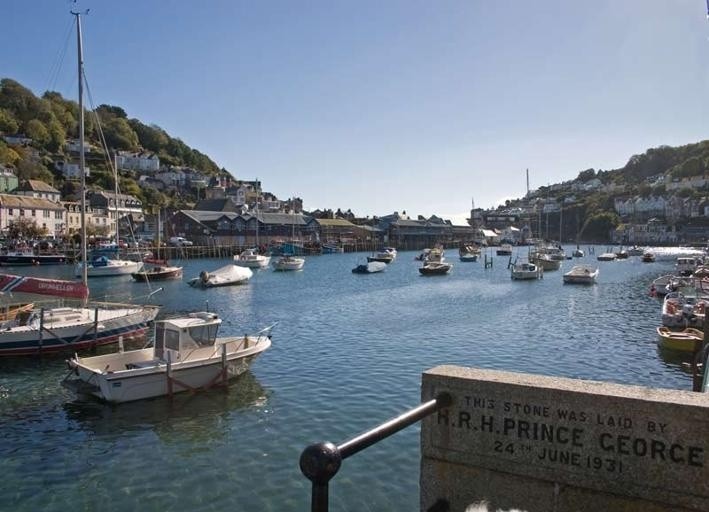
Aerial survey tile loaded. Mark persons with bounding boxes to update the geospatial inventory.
[0,237,168,253]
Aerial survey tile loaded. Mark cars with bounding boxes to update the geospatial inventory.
[31,239,55,250]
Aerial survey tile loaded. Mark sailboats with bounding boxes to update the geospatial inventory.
[233,177,272,267]
[72,149,148,283]
[0,0,159,359]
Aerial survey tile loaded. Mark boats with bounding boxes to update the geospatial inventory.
[366,246,398,264]
[130,259,184,282]
[65,302,281,408]
[186,263,254,291]
[510,262,544,280]
[272,253,306,272]
[646,246,709,352]
[0,250,69,267]
[351,260,388,273]
[563,263,600,285]
[415,169,660,275]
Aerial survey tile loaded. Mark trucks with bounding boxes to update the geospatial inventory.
[168,236,194,246]
